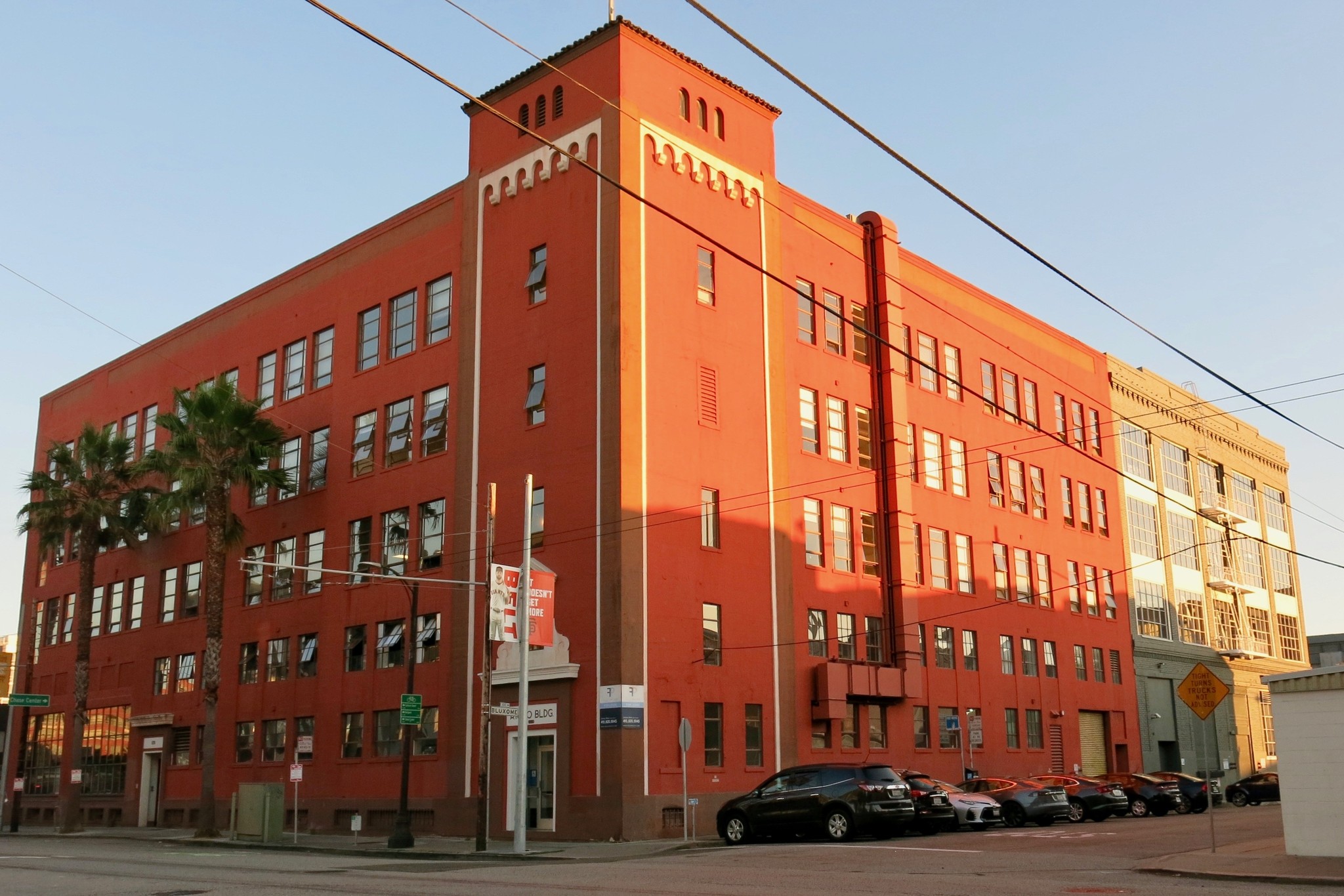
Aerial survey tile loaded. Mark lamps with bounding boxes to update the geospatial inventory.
[1151,713,1161,719]
[1159,662,1166,666]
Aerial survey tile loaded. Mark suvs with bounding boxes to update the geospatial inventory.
[716,762,917,846]
[893,768,956,835]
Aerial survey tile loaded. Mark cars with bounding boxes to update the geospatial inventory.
[1225,771,1281,808]
[1025,773,1129,824]
[1127,771,1223,815]
[930,779,1003,832]
[952,776,1072,829]
[1089,772,1183,818]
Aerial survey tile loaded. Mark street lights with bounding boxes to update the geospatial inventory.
[358,561,421,849]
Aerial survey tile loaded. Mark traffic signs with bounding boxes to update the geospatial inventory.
[8,694,50,707]
[400,703,422,711]
[400,716,421,725]
[400,710,422,718]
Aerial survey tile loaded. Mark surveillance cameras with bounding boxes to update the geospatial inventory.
[1155,713,1161,718]
